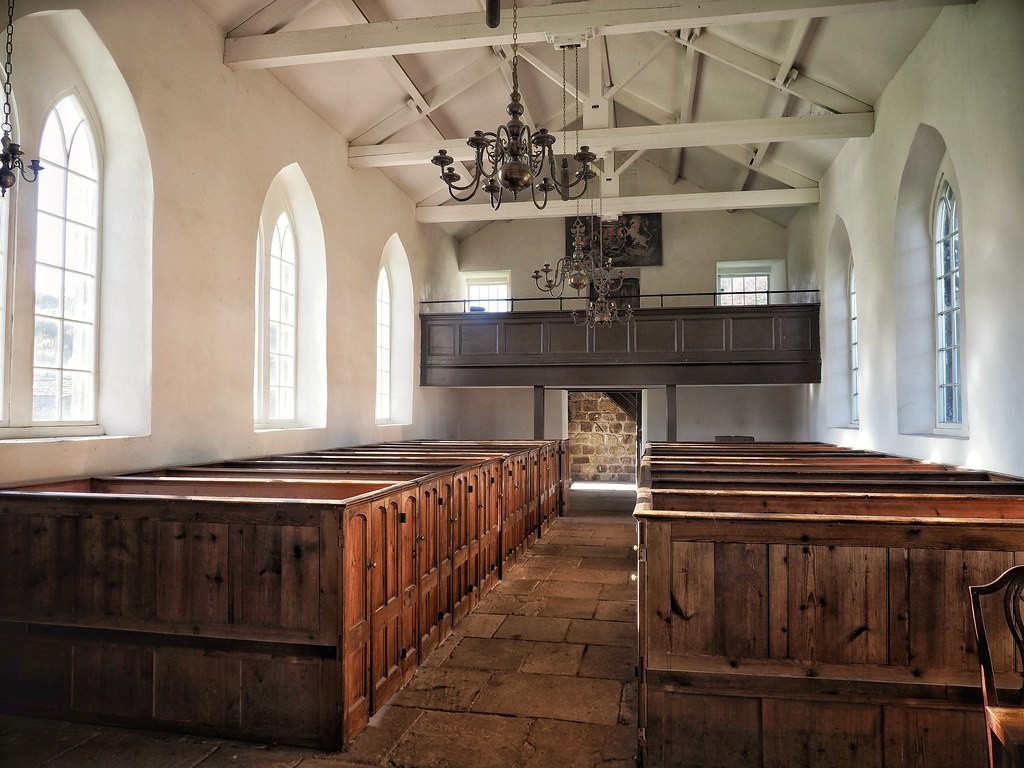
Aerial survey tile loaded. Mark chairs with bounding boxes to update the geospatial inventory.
[968,564,1024,768]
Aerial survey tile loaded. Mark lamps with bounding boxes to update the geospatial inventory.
[0,0,45,197]
[530,43,634,329]
[430,0,597,211]
